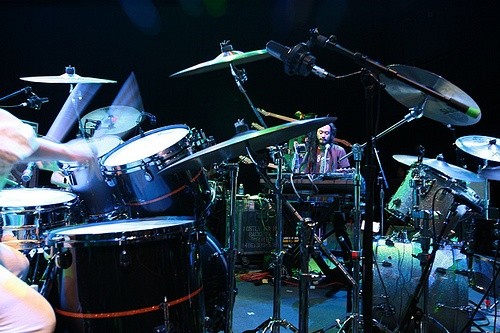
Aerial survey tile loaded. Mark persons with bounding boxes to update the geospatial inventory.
[0,105,102,333]
[286,121,355,272]
[0,239,31,281]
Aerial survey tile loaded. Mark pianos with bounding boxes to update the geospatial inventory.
[259,170,366,285]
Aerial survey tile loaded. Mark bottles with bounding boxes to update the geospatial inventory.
[238,183,244,195]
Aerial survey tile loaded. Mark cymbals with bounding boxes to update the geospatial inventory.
[455,135,500,162]
[479,166,500,181]
[392,154,484,183]
[379,63,482,127]
[79,105,142,133]
[156,116,339,176]
[20,72,116,84]
[169,48,273,77]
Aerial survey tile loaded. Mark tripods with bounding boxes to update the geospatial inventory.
[316,95,392,333]
[245,151,304,333]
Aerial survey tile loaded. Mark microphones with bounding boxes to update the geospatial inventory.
[25,97,48,108]
[446,187,484,214]
[264,41,337,80]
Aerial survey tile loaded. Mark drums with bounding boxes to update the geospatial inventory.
[1,187,80,251]
[45,219,206,333]
[385,162,481,242]
[56,135,127,219]
[100,124,216,221]
[205,232,238,333]
[370,235,471,333]
